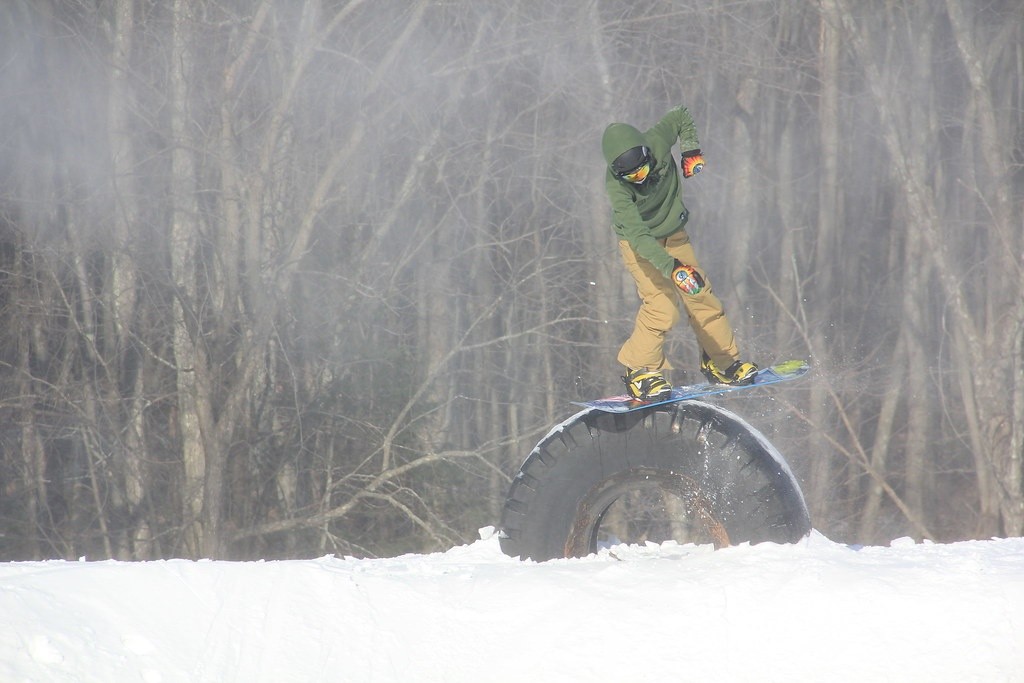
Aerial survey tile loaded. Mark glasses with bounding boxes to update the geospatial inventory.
[618,160,651,183]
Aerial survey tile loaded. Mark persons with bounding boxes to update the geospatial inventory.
[602,105,758,401]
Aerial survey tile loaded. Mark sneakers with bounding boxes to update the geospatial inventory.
[700,349,759,386]
[625,366,672,400]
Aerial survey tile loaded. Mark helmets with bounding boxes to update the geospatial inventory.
[611,146,650,176]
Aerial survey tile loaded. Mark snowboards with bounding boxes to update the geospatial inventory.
[568,359,811,416]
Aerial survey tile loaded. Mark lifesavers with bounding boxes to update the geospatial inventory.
[494,395,813,563]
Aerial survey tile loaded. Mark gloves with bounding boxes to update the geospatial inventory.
[670,259,706,295]
[681,150,706,178]
